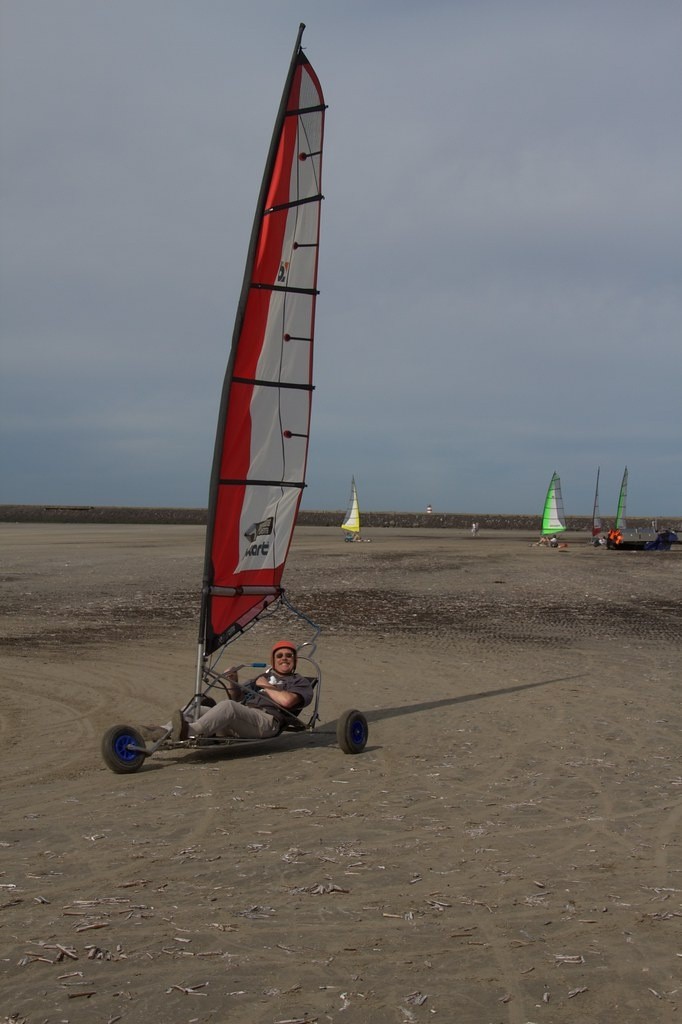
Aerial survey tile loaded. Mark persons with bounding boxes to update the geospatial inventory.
[536,535,557,547]
[135,640,314,743]
[592,536,608,545]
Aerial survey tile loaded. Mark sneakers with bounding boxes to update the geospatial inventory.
[137,724,168,741]
[171,709,188,742]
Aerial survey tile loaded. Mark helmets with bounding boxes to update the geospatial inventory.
[273,642,296,672]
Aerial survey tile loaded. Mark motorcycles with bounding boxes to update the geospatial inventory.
[100,639,369,774]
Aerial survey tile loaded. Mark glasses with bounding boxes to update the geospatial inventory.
[276,653,294,658]
